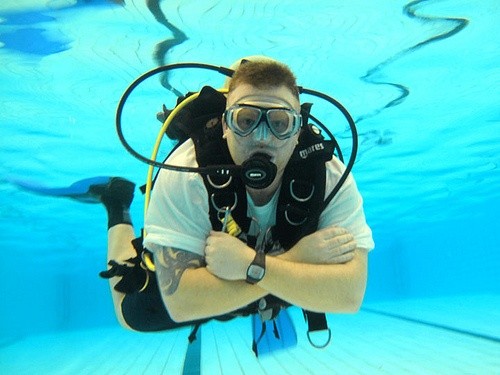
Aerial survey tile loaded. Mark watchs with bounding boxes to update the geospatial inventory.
[243,249,268,286]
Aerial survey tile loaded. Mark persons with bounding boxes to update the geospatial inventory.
[88,54,374,335]
